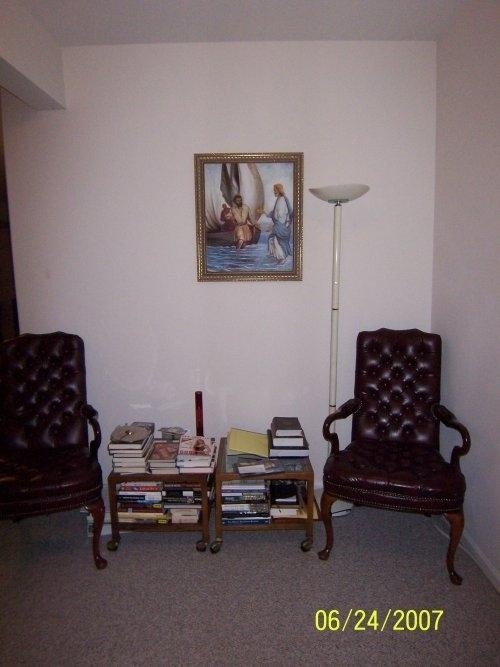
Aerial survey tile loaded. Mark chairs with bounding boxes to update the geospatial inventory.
[317,326,472,588]
[0,330,109,571]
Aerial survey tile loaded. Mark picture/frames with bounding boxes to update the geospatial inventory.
[193,150,305,282]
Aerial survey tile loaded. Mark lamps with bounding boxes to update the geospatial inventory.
[310,183,371,516]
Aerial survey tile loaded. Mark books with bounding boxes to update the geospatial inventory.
[87,415,354,540]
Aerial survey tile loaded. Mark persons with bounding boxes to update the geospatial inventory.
[255,182,293,266]
[227,194,261,251]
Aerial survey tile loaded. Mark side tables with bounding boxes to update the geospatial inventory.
[105,433,313,555]
